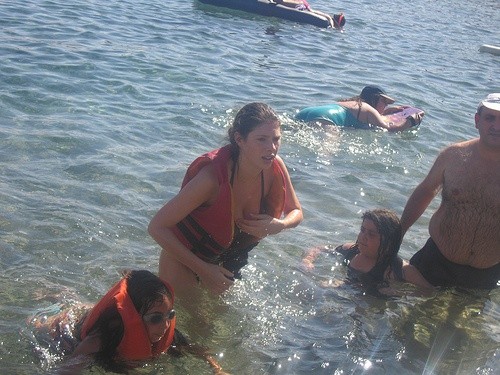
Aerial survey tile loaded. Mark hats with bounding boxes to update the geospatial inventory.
[361,85,395,104]
[478,92,500,111]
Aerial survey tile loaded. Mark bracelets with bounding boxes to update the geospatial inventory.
[406,115,417,127]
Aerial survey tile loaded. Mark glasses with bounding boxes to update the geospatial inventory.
[141,309,176,323]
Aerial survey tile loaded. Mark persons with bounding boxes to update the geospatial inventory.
[294,84,424,132]
[27,269,228,375]
[147,102,303,304]
[400,93,500,292]
[304,208,436,297]
